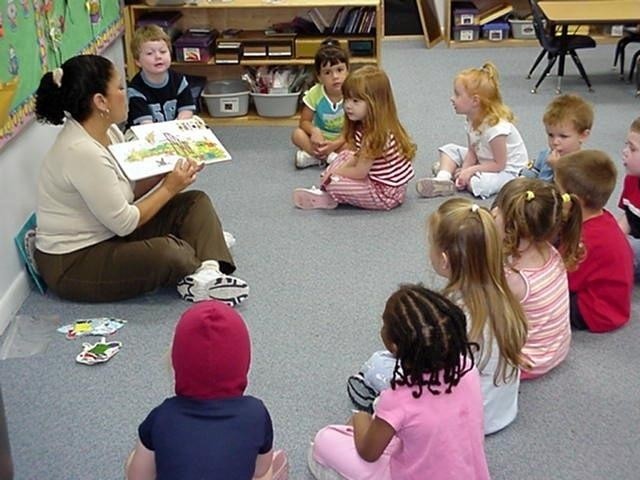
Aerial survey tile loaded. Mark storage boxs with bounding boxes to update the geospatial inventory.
[174,26,218,63]
[450,8,510,41]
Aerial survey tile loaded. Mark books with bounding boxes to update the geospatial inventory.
[478,3,514,26]
[13,211,48,296]
[129,115,206,140]
[109,124,228,180]
[136,5,376,66]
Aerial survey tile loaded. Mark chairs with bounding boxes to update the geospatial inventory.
[528,0,597,98]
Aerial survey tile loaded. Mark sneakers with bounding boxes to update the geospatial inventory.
[346,375,379,410]
[292,186,338,209]
[307,442,344,479]
[432,161,442,175]
[295,150,322,169]
[222,231,236,250]
[176,269,251,306]
[415,178,456,198]
[261,450,289,479]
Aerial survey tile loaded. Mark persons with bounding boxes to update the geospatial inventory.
[490,178,582,379]
[293,66,414,213]
[527,94,593,181]
[345,200,529,434]
[292,40,349,169]
[32,52,250,310]
[417,58,528,198]
[124,22,199,126]
[123,298,291,480]
[550,150,633,334]
[308,280,494,480]
[618,118,640,273]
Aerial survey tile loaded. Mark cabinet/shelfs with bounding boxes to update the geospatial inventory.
[445,0,640,48]
[123,0,384,126]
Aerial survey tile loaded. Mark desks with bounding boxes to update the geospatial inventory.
[537,0,640,95]
